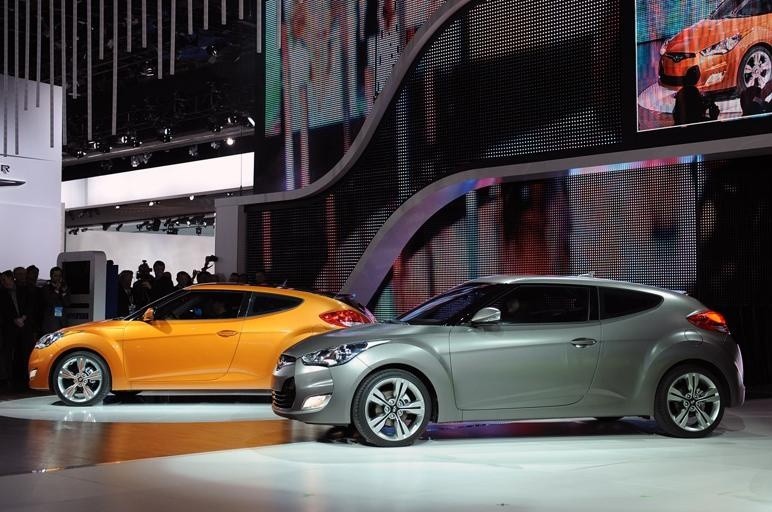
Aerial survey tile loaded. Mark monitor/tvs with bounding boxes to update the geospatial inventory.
[62,260,90,294]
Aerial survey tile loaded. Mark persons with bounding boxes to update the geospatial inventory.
[119,261,266,318]
[740,79,772,116]
[287,1,401,130]
[0,265,72,401]
[672,65,715,124]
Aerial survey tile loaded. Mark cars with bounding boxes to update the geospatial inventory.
[268,269,747,447]
[27,281,377,408]
[658,1,771,98]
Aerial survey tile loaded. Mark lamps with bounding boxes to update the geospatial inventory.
[63,29,258,168]
